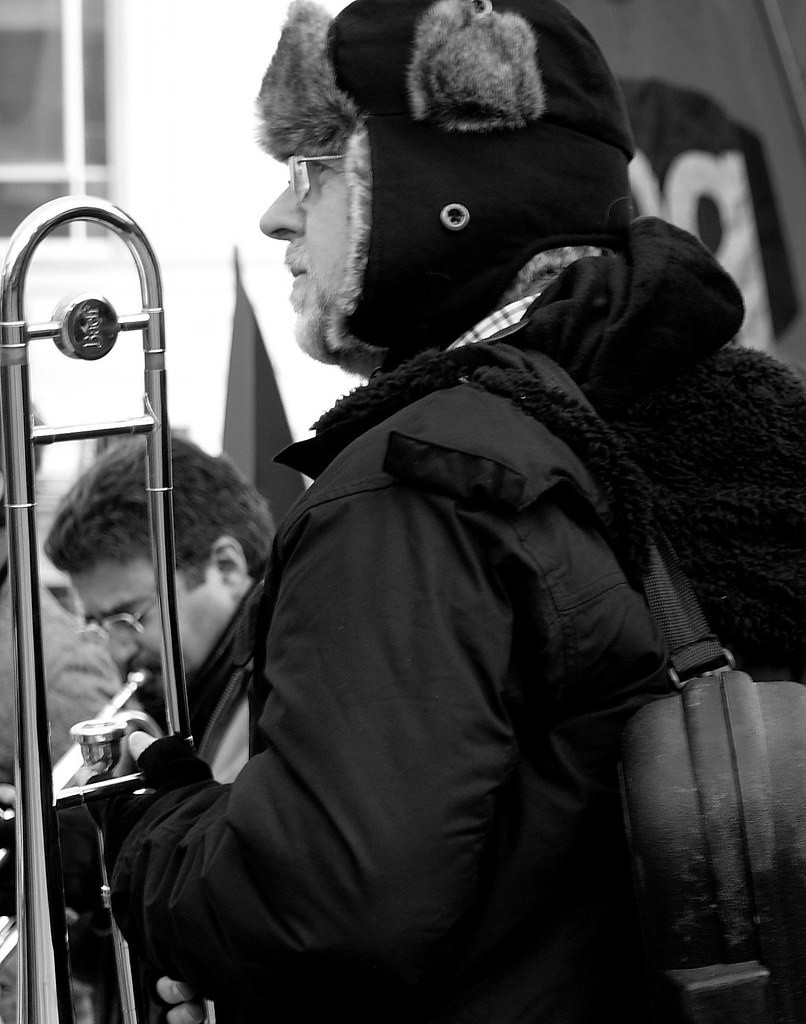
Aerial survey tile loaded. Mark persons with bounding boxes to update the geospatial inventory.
[45,437,274,750]
[87,1,804,1022]
[0,401,143,803]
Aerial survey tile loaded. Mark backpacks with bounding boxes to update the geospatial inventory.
[491,333,806,1024]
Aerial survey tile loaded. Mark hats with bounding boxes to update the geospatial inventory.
[256,0,635,362]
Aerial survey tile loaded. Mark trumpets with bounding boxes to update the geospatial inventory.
[0,667,166,964]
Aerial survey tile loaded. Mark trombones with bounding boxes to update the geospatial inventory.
[0,194,217,1024]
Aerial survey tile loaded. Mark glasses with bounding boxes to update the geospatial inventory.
[287,153,348,206]
[75,558,189,648]
